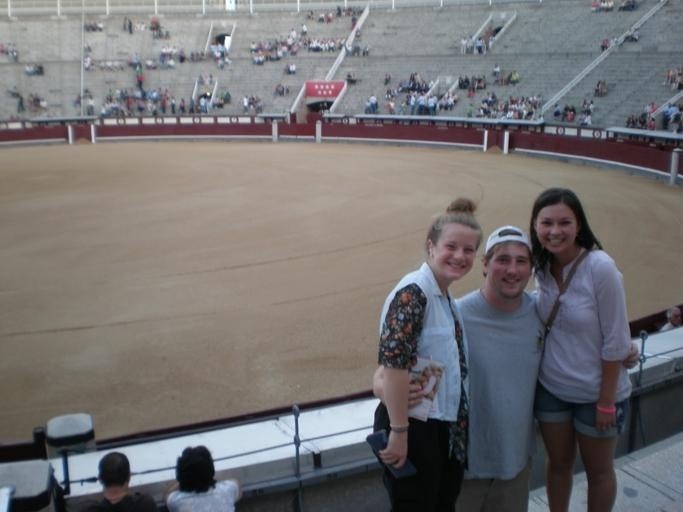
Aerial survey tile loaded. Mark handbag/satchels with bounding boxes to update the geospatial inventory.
[365,429,418,480]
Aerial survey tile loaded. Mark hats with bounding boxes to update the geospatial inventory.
[484,224,530,255]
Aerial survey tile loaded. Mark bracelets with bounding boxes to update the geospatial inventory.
[388,422,410,434]
[595,405,617,416]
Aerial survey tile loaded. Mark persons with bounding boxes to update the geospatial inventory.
[161,443,242,512]
[0,0,681,146]
[78,451,158,511]
[527,187,634,511]
[365,196,485,511]
[658,306,682,333]
[370,220,644,512]
